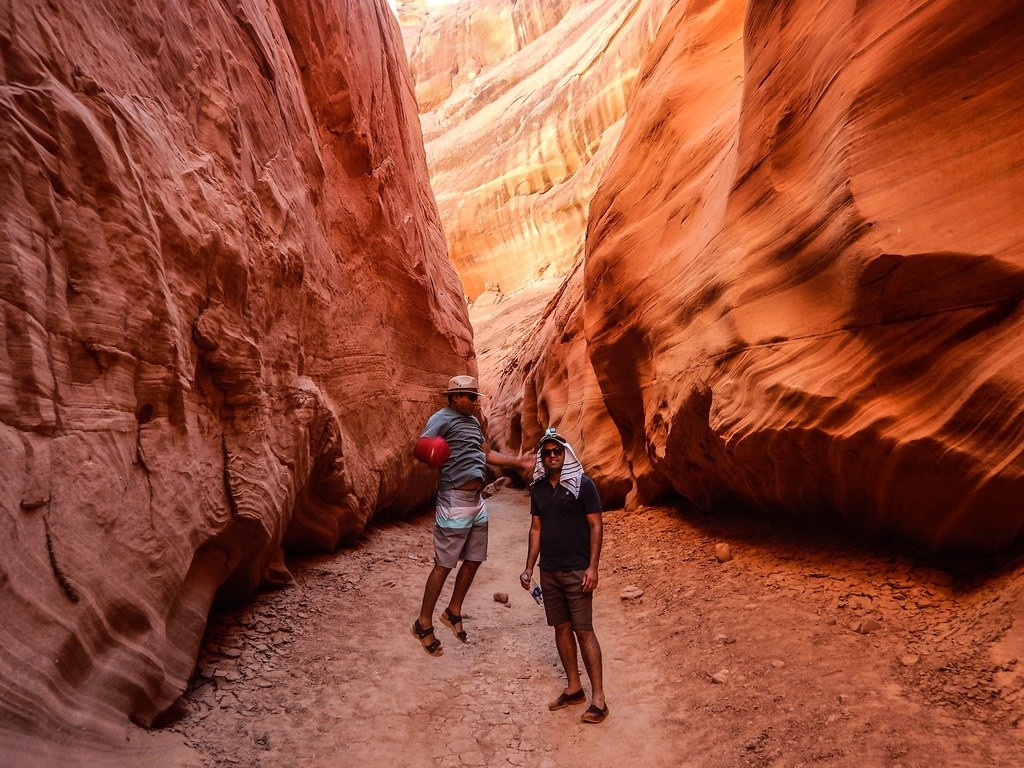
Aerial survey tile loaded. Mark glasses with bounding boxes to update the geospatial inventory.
[459,392,477,401]
[541,446,564,457]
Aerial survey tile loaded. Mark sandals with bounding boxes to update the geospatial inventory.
[440,607,470,644]
[410,619,443,656]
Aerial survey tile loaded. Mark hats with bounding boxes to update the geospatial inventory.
[442,375,486,397]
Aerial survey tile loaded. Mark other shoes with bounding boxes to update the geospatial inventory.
[548,688,586,711]
[582,701,610,723]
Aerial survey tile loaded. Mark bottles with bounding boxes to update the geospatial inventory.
[523,574,545,607]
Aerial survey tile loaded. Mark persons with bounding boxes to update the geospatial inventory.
[520,429,609,724]
[409,376,537,657]
